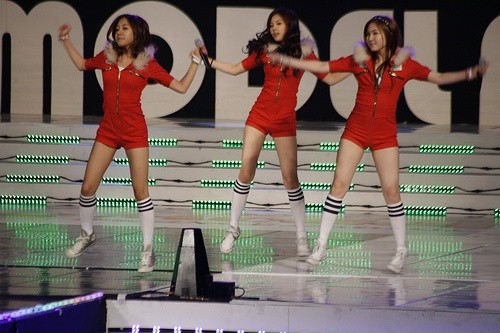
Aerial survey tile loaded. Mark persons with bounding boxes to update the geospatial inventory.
[267,16,490,274]
[190,10,354,262]
[58,14,208,273]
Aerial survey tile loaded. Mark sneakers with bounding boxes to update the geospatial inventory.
[220,227,241,253]
[137,244,157,272]
[387,250,408,273]
[306,245,328,265]
[296,237,311,259]
[66,229,97,258]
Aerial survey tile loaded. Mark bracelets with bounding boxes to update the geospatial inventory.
[192,52,201,64]
[58,35,70,40]
[464,64,476,81]
[210,58,214,67]
[279,55,293,67]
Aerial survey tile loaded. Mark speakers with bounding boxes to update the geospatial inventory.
[170,227,214,300]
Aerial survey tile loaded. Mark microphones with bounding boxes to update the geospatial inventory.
[194,38,212,72]
